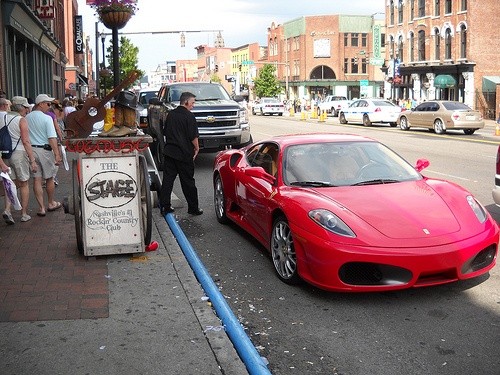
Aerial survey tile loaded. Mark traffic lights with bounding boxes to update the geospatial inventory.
[239,64,242,72]
[215,65,218,72]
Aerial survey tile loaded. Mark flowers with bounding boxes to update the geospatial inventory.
[86,0,139,13]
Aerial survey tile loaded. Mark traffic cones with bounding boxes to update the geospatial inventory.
[494,123,500,136]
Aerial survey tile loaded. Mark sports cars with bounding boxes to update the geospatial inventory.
[212,132,500,293]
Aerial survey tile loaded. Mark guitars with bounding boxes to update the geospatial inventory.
[63,71,138,138]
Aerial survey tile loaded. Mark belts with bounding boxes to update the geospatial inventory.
[31,145,45,148]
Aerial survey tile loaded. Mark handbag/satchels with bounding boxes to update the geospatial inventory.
[0,167,23,211]
[61,144,70,171]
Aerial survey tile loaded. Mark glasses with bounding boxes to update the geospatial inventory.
[46,102,52,106]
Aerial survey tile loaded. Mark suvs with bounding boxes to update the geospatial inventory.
[146,80,254,171]
[316,95,348,117]
[492,145,500,208]
[135,89,183,130]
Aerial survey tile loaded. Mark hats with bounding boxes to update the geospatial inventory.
[12,96,32,108]
[110,91,145,112]
[35,94,56,106]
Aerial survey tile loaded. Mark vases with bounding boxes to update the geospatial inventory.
[98,13,134,29]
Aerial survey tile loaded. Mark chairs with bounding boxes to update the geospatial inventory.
[325,155,360,185]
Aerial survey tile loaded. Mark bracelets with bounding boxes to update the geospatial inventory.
[29,158,35,164]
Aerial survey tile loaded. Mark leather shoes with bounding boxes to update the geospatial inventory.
[160,206,175,215]
[187,208,204,215]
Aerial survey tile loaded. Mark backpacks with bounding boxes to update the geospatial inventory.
[0,114,22,159]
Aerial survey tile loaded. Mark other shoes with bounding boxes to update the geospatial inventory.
[41,181,59,188]
[20,215,32,222]
[47,201,63,212]
[36,208,47,217]
[2,209,16,225]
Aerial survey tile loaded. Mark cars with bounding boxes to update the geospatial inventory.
[252,98,284,116]
[398,101,485,135]
[338,99,407,127]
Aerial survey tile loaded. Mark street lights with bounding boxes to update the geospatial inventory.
[101,29,107,98]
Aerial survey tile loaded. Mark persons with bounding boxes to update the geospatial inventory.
[0,94,84,225]
[159,92,204,215]
[283,96,320,112]
[393,99,418,111]
